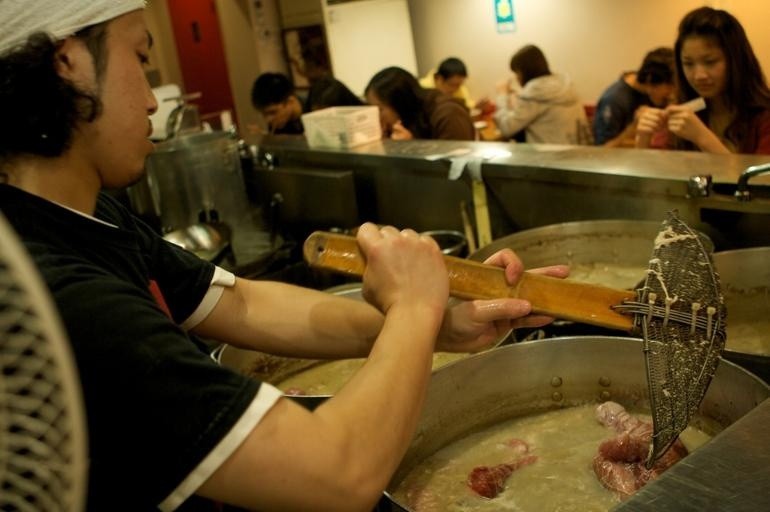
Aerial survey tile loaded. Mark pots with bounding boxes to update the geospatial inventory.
[214,278,515,404]
[384,339,770,511]
[712,247,768,360]
[462,218,713,291]
[146,131,250,233]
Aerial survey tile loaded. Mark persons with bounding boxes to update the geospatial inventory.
[364,66,476,144]
[418,57,490,117]
[251,72,311,135]
[295,36,366,114]
[495,44,589,144]
[638,6,769,155]
[593,45,677,146]
[0,1,568,510]
